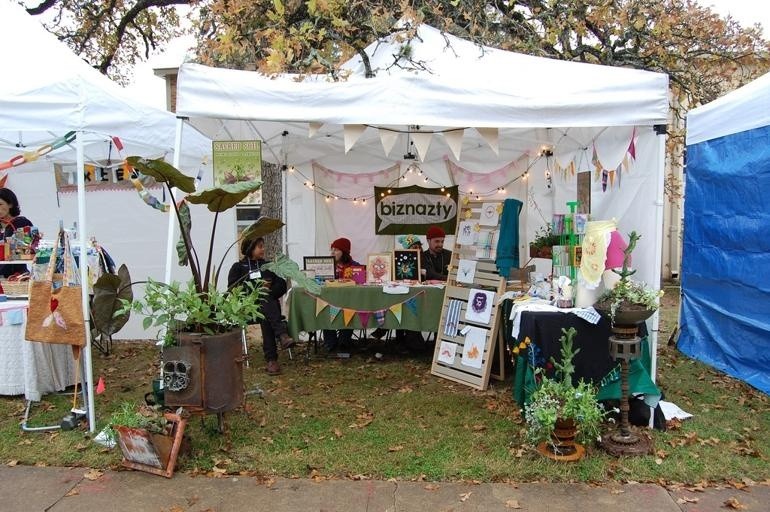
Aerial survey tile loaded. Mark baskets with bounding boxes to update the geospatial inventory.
[2,275,30,295]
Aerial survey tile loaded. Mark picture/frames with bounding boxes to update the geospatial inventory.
[365,252,391,283]
[393,249,421,282]
[303,256,336,282]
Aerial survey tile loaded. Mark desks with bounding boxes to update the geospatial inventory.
[287,280,446,366]
[500,291,662,422]
[0,260,86,402]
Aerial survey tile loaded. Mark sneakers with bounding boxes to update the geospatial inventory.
[372,329,388,338]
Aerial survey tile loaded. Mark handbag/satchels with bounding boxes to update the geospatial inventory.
[25,231,87,347]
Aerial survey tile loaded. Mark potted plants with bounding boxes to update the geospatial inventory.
[110,399,191,479]
[529,223,577,259]
[111,154,321,434]
[592,230,664,456]
[524,327,620,462]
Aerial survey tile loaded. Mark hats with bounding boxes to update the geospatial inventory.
[331,239,351,253]
[398,235,421,249]
[241,233,264,255]
[426,225,447,240]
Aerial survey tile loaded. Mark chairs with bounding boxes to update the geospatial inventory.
[318,329,367,351]
[385,329,431,350]
[242,315,293,368]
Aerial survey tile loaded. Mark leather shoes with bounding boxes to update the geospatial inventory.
[267,358,281,375]
[280,334,294,348]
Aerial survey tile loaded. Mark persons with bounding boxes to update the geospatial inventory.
[323,238,361,353]
[0,187,40,279]
[371,234,426,346]
[226,236,295,374]
[421,224,452,282]
